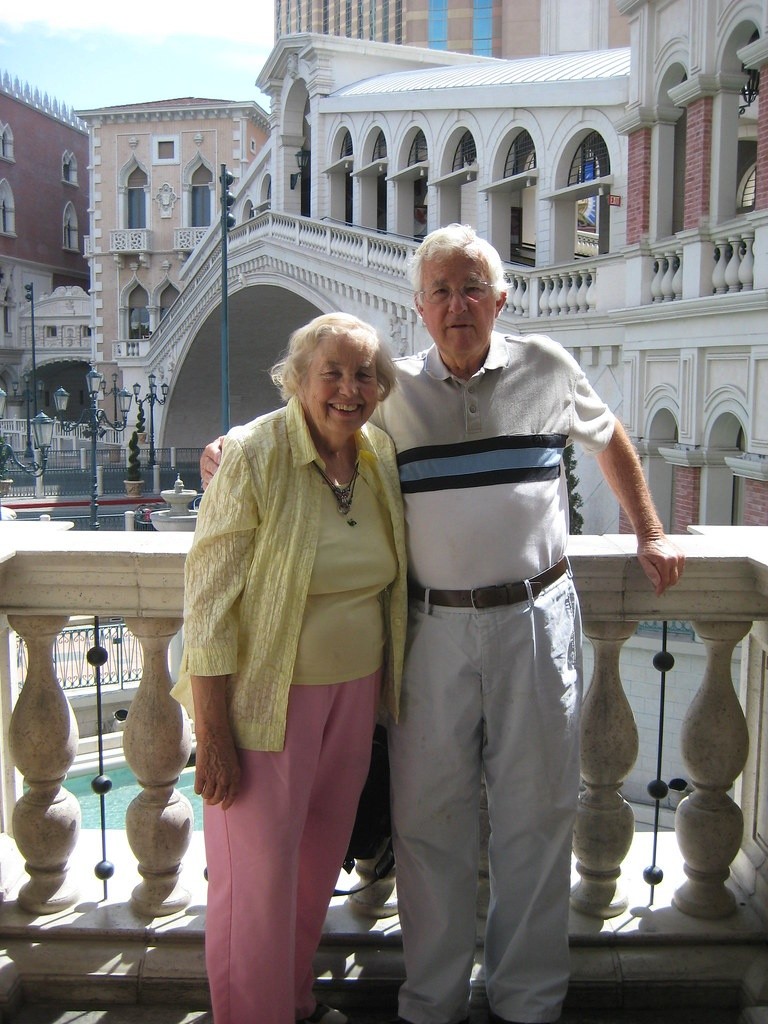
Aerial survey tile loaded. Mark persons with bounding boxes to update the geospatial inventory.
[199,217,688,1023]
[162,307,412,1024]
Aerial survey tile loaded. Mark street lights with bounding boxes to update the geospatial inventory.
[54,367,133,531]
[111,373,118,427]
[0,388,58,526]
[219,162,237,436]
[12,381,19,397]
[23,373,34,457]
[134,373,168,469]
[25,281,38,417]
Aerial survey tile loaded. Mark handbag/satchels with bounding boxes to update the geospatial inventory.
[333,723,394,897]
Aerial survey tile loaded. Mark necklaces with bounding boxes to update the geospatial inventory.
[310,459,359,526]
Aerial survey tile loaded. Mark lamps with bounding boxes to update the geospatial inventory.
[739,64,761,118]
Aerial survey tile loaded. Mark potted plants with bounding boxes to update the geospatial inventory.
[0,437,13,497]
[123,430,145,497]
[136,398,147,443]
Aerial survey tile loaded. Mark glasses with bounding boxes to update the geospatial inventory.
[420,282,494,304]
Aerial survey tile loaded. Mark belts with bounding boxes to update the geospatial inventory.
[407,556,569,610]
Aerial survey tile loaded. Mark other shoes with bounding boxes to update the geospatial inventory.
[305,1005,355,1024]
